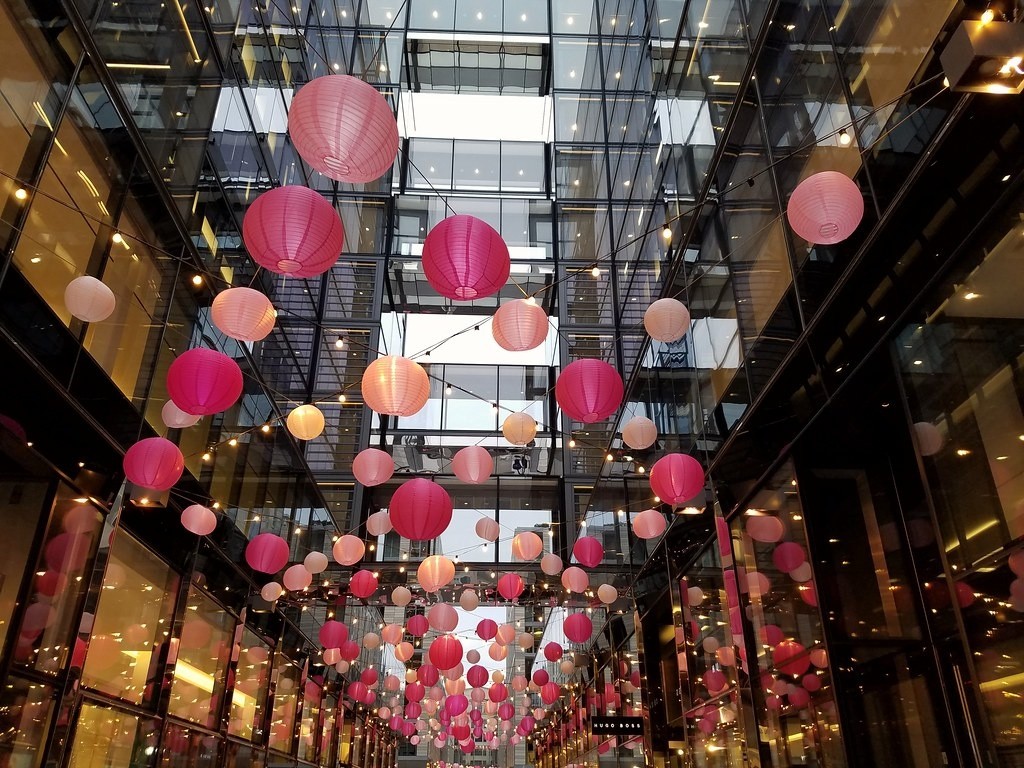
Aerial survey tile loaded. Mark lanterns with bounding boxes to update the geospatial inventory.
[60,75,863,751]
[525,421,1024,768]
[0,503,391,768]
[425,760,480,768]
